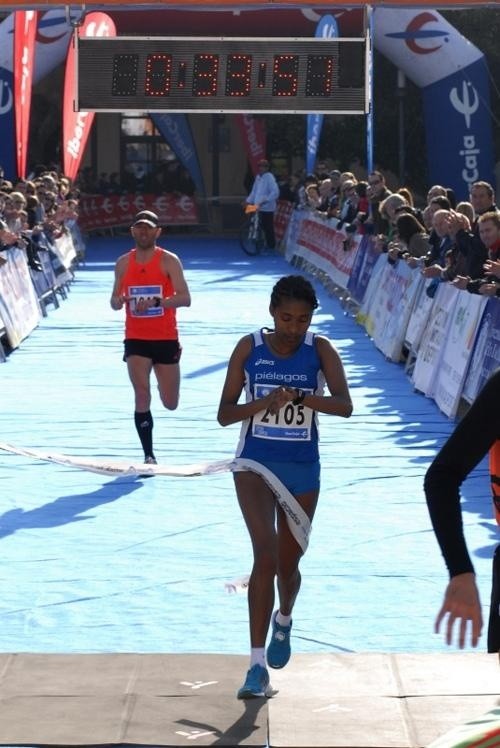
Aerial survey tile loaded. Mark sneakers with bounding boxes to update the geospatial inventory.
[27,245,48,272]
[139,457,157,477]
[237,663,269,698]
[266,610,293,669]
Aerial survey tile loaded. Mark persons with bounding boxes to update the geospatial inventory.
[239,160,281,256]
[217,275,352,699]
[98,157,197,237]
[424,368,500,659]
[284,160,500,298]
[111,210,191,480]
[0,162,81,254]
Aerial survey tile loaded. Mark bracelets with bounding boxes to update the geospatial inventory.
[153,296,160,307]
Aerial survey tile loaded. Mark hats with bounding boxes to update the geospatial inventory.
[133,210,158,228]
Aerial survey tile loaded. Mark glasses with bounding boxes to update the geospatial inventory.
[368,180,380,185]
[0,184,53,204]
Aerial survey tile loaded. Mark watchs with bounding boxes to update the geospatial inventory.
[292,388,306,407]
